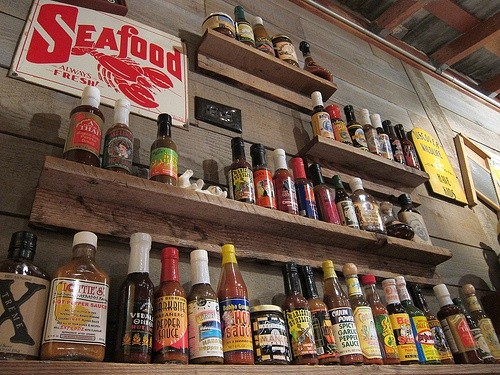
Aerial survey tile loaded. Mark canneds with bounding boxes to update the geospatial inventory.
[201,12,235,40]
[251,304,292,365]
[271,34,299,68]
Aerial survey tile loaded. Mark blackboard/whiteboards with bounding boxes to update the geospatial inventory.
[464,154,500,210]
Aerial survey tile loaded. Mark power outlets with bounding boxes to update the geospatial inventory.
[194,95,243,134]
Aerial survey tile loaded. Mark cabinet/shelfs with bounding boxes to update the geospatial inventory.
[0,27,500,375]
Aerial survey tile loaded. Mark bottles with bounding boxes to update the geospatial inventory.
[311,91,420,170]
[299,41,333,83]
[308,164,341,225]
[281,261,341,365]
[288,158,320,220]
[186,249,224,365]
[217,244,254,365]
[226,137,256,205]
[433,284,500,365]
[349,178,387,235]
[0,231,52,361]
[234,6,275,57]
[382,276,455,364]
[343,262,400,365]
[153,247,189,364]
[381,193,432,246]
[272,148,299,216]
[101,99,134,175]
[250,143,277,210]
[62,86,105,168]
[331,175,359,230]
[114,233,157,364]
[321,260,364,365]
[149,113,178,186]
[40,231,111,362]
